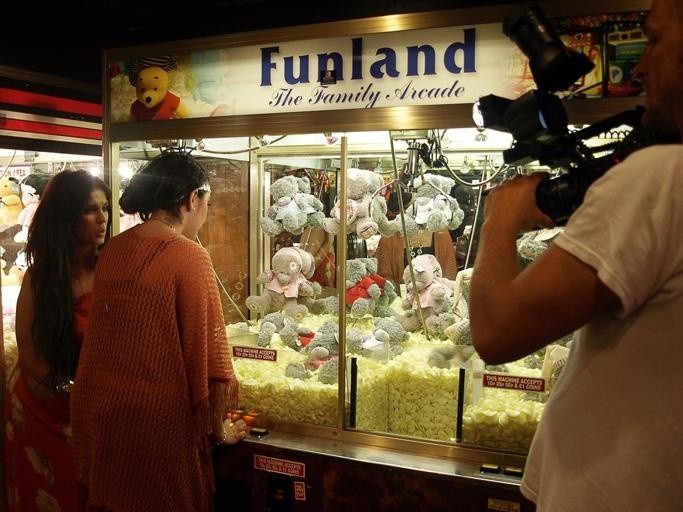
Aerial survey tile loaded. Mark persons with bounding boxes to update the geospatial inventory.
[0,167,112,511]
[66,154,247,511]
[471,0,682,511]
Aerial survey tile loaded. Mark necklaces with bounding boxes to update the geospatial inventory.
[150,214,176,232]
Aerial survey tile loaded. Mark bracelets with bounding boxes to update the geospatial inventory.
[211,425,228,446]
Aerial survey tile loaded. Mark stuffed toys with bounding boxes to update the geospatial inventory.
[316,169,465,240]
[259,175,323,236]
[244,246,410,385]
[0,173,53,333]
[516,228,553,262]
[126,67,192,121]
[393,251,478,368]
[245,168,572,402]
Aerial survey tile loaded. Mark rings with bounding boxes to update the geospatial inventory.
[228,422,234,428]
